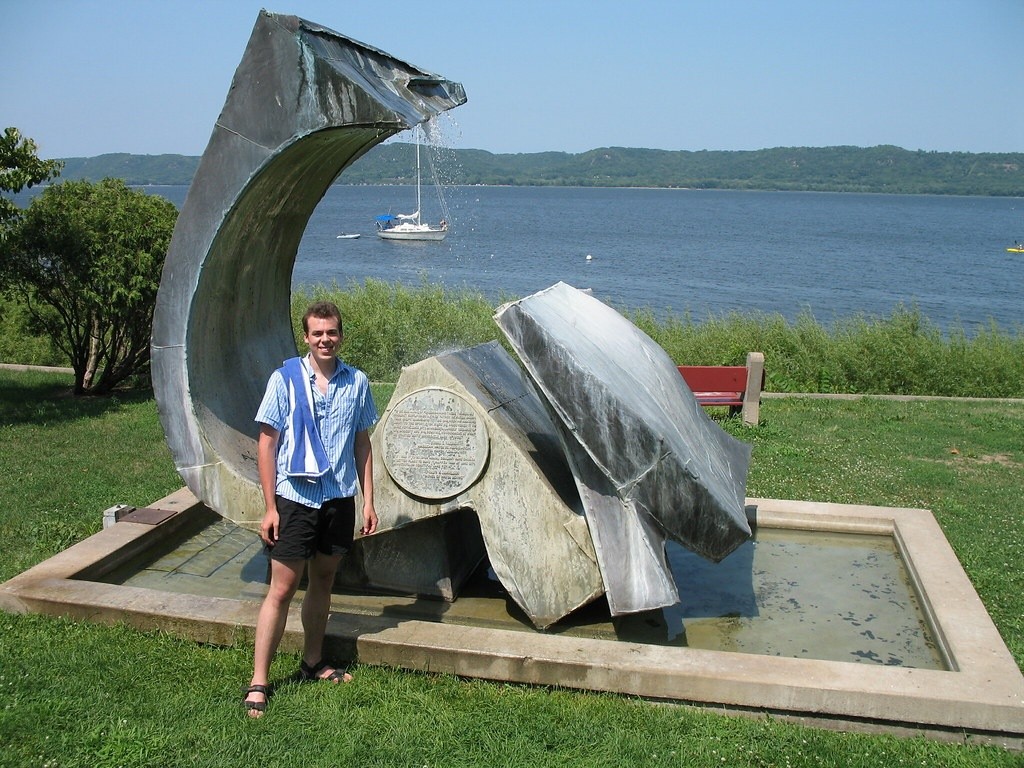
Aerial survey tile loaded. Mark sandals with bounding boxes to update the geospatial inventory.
[299,657,352,685]
[244,685,267,719]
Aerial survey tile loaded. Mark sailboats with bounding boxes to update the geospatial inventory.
[372,125,449,241]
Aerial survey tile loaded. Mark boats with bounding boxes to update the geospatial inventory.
[337,233,361,240]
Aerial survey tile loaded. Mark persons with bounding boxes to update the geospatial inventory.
[386,220,392,226]
[246,302,379,718]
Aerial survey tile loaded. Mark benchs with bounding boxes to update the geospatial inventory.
[677,352,767,428]
